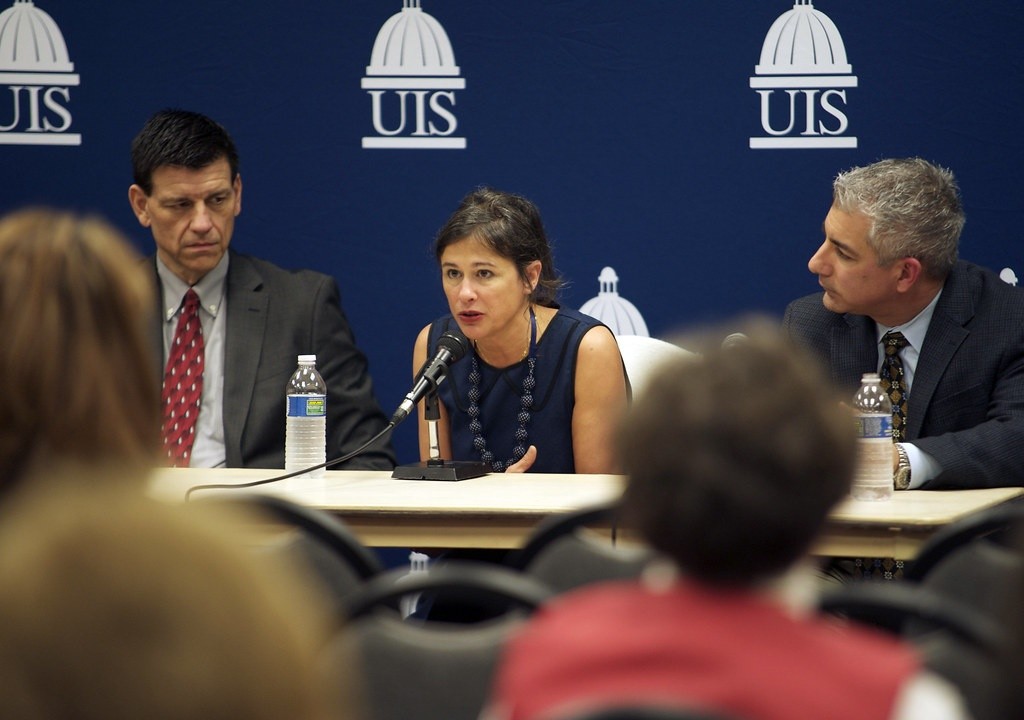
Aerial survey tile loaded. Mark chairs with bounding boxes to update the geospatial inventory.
[196,491,1024,720]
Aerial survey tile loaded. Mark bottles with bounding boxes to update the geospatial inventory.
[285,355,326,478]
[852,374,893,501]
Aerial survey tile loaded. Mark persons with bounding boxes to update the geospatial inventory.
[128,109,396,471]
[779,157,1024,627]
[473,342,924,720]
[0,471,338,720]
[413,188,633,627]
[0,210,159,508]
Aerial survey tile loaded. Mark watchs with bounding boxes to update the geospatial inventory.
[893,444,911,490]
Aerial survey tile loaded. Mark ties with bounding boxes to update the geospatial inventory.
[878,331,911,444]
[160,288,205,467]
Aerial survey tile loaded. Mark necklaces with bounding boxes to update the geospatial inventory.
[468,316,538,475]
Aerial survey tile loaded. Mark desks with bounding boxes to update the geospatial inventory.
[155,454,1024,567]
